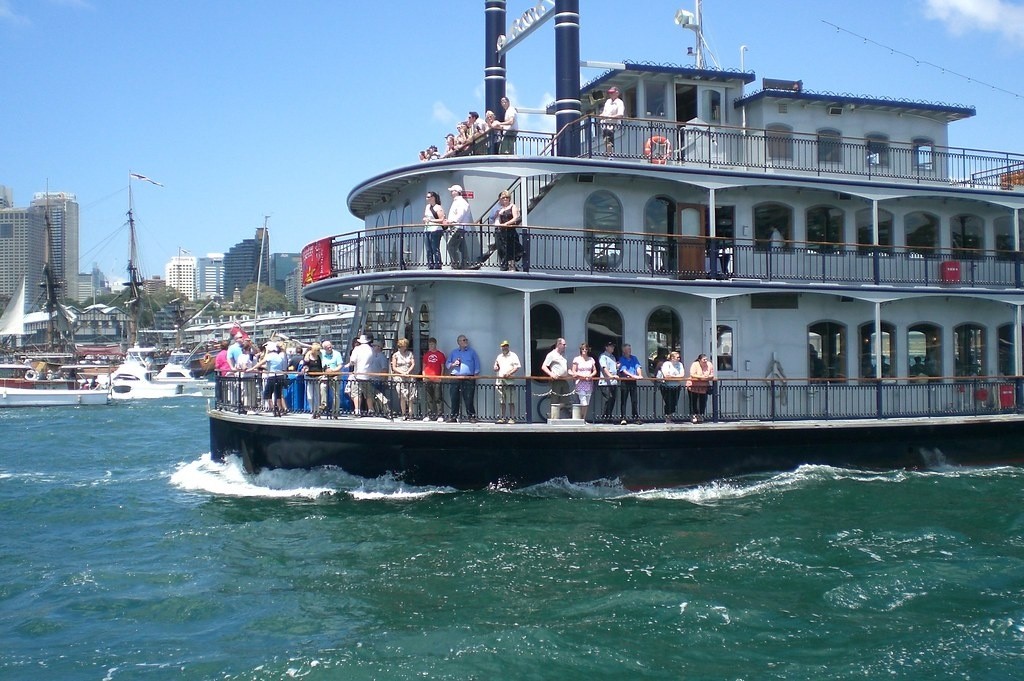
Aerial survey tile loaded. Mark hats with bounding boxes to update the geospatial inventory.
[605,341,617,347]
[448,185,462,194]
[500,341,510,347]
[357,335,370,344]
[266,341,278,351]
[608,87,620,93]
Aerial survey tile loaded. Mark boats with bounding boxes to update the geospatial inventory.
[0,317,224,382]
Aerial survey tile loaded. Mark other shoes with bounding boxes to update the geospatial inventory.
[428,265,434,269]
[446,418,457,422]
[634,420,642,424]
[621,420,627,425]
[470,418,477,423]
[508,419,515,424]
[691,417,697,423]
[497,420,503,423]
[319,405,326,410]
[698,417,704,423]
[434,264,442,269]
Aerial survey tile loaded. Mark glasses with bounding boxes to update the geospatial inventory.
[463,339,468,342]
[469,117,474,119]
[502,197,509,199]
[427,195,433,198]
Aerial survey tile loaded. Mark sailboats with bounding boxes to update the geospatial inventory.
[97,168,216,401]
[0,178,114,408]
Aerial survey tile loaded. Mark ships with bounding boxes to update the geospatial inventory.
[204,0,1024,498]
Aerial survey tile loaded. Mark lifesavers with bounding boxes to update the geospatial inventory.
[204,354,210,364]
[24,369,36,380]
[644,135,673,164]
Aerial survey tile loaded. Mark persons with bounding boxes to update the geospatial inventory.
[864,354,889,377]
[542,338,577,409]
[660,352,685,422]
[447,184,470,269]
[494,341,521,424]
[769,224,785,247]
[446,335,480,422]
[599,341,643,424]
[957,370,977,380]
[686,353,714,422]
[598,87,625,154]
[911,356,935,376]
[498,96,520,154]
[571,343,597,420]
[423,191,445,270]
[84,375,98,390]
[216,333,388,420]
[810,343,840,384]
[489,190,523,271]
[445,111,501,154]
[392,338,418,421]
[422,337,446,422]
[419,147,439,161]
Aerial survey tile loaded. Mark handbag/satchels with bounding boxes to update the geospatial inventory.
[707,386,714,395]
[442,225,448,230]
